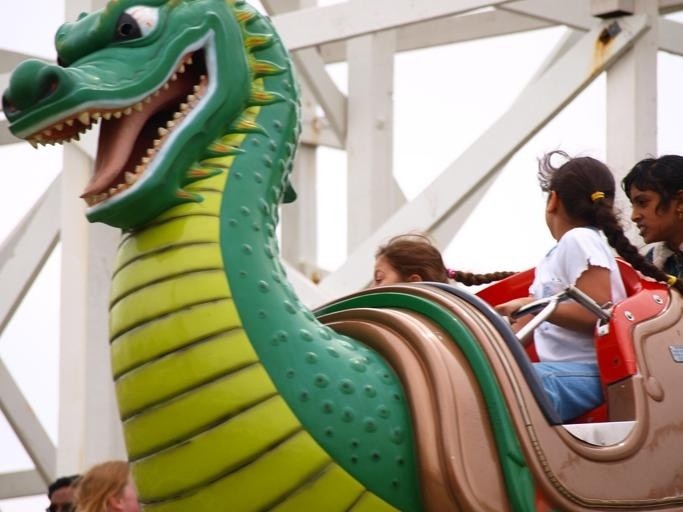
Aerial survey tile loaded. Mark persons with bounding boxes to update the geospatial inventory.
[613,151,683,297]
[68,459,141,512]
[43,473,84,511]
[372,233,522,291]
[490,146,683,423]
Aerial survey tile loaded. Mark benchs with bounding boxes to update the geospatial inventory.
[475,256,671,427]
